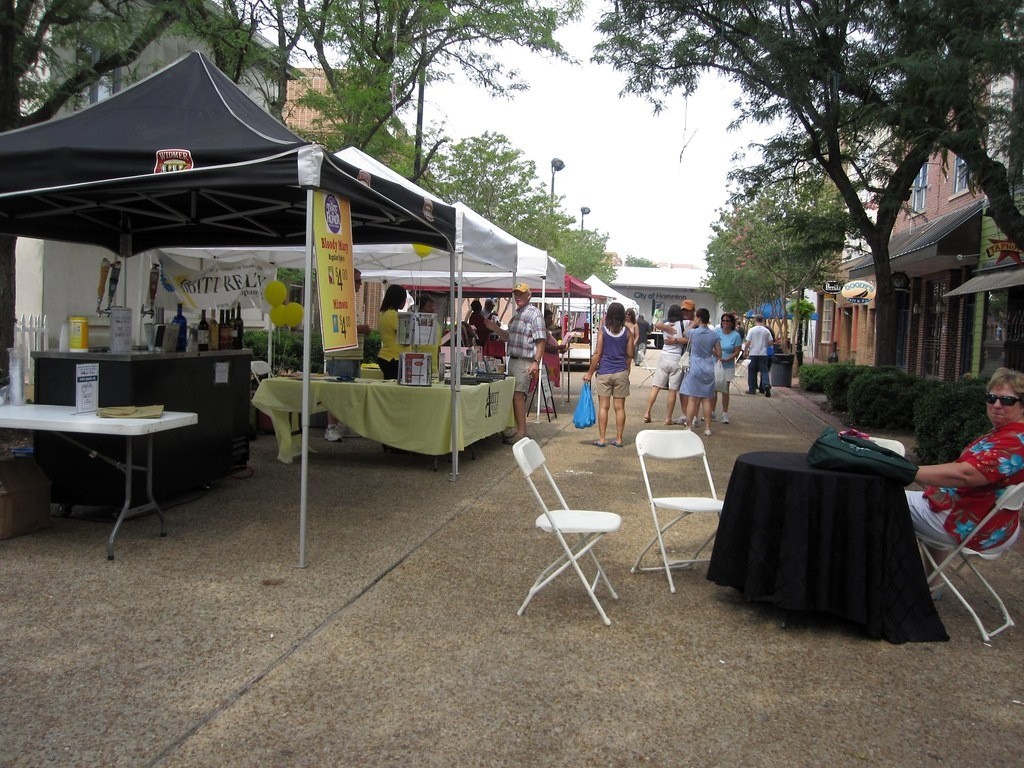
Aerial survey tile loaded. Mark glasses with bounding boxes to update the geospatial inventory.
[355,279,361,285]
[722,320,732,322]
[986,394,1021,406]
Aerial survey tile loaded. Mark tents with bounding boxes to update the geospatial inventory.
[0,49,641,573]
[744,296,820,320]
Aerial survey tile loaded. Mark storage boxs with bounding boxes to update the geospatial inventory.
[0,458,52,540]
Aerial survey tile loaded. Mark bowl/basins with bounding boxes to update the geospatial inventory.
[144,324,179,352]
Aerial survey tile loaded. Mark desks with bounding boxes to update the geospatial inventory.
[706,453,950,644]
[0,404,198,560]
[254,375,516,464]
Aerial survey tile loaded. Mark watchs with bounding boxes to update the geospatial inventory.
[674,337,678,344]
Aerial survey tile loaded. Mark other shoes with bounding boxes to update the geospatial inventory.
[704,429,711,436]
[504,433,526,444]
[701,411,716,421]
[764,385,771,397]
[721,413,729,424]
[673,415,686,423]
[692,415,698,427]
[541,407,554,413]
[745,390,755,394]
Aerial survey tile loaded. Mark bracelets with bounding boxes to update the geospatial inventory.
[534,359,540,363]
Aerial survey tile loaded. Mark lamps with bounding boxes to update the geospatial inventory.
[935,299,950,315]
[913,301,925,314]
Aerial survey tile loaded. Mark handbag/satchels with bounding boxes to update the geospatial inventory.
[573,383,595,428]
[808,428,918,486]
[677,351,692,369]
[713,359,728,394]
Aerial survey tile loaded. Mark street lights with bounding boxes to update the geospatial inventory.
[549,158,566,214]
[581,207,591,231]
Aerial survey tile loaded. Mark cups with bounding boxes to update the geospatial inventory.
[498,364,505,374]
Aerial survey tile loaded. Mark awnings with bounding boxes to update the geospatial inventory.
[941,265,1024,298]
[848,199,982,277]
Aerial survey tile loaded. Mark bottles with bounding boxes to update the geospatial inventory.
[171,303,243,351]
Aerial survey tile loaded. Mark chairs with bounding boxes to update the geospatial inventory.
[729,359,751,396]
[630,428,725,593]
[913,483,1024,642]
[513,437,623,626]
[251,361,276,384]
[638,351,658,387]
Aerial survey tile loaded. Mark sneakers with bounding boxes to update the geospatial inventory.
[325,426,342,442]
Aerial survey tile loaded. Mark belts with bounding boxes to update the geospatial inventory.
[510,356,518,359]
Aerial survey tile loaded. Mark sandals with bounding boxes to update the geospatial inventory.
[644,416,651,422]
[664,420,674,425]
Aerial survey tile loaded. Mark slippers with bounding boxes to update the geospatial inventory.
[593,440,606,447]
[611,441,623,446]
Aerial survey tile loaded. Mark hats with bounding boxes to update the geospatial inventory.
[681,300,695,310]
[512,283,530,292]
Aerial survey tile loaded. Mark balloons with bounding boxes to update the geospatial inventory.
[264,280,287,306]
[412,242,433,259]
[268,305,286,328]
[281,302,304,328]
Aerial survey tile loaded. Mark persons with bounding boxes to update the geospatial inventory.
[468,300,498,348]
[411,294,443,374]
[678,308,722,437]
[480,299,502,341]
[483,282,547,445]
[701,313,742,424]
[376,284,413,381]
[644,297,777,437]
[634,314,651,366]
[324,267,372,443]
[529,309,570,414]
[582,302,634,447]
[743,314,774,398]
[905,367,1024,602]
[623,308,640,377]
